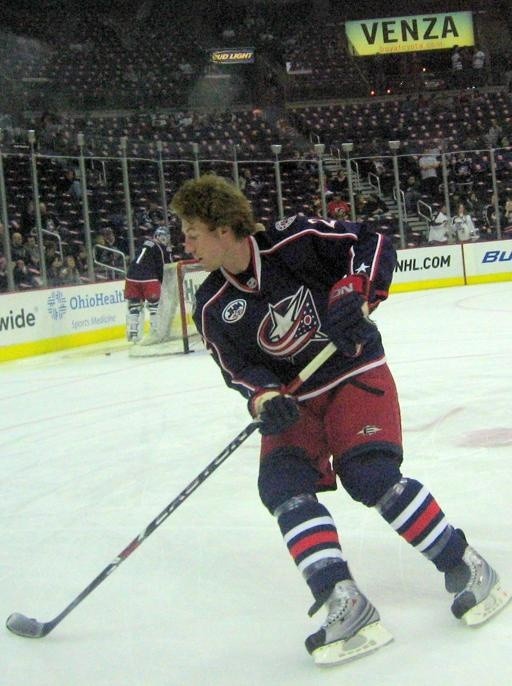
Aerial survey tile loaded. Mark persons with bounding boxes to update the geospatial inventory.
[469,44,485,88]
[168,174,503,655]
[449,44,466,90]
[1,88,510,293]
[123,225,173,347]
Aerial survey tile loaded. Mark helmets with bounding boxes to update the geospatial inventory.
[153,224,172,248]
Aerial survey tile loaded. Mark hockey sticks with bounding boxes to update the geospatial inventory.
[6,340,338,637]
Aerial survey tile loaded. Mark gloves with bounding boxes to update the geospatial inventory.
[324,273,380,360]
[245,385,299,436]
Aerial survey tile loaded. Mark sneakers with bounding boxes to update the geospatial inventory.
[449,544,498,620]
[305,576,380,655]
